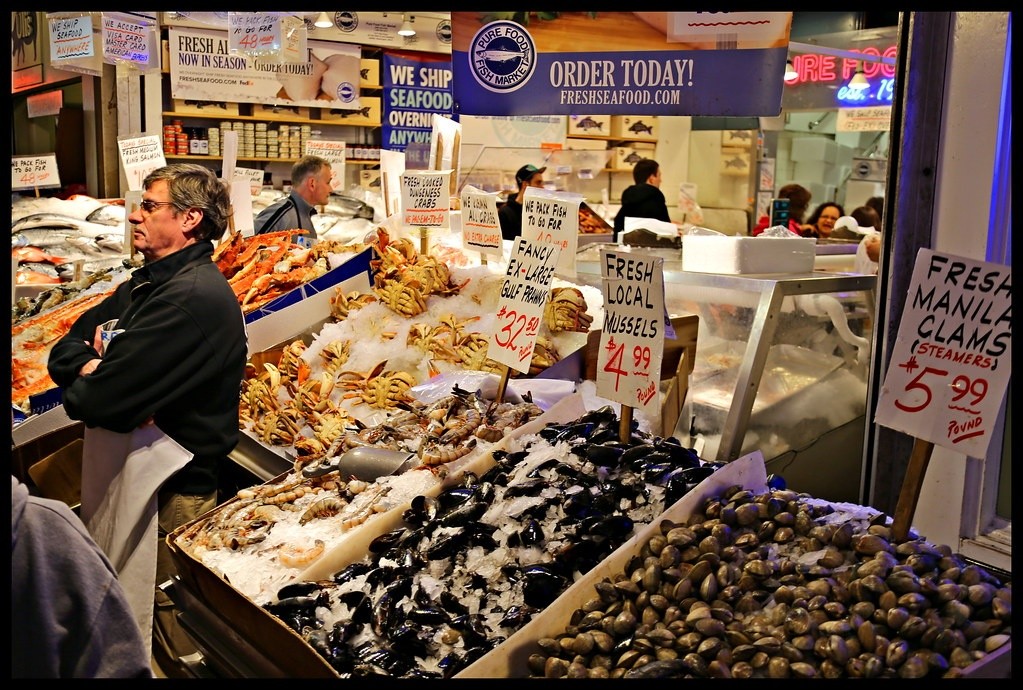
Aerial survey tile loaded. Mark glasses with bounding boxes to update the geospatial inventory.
[139,197,184,219]
[821,214,836,223]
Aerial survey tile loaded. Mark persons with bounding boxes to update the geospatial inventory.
[850,196,884,231]
[48,160,248,668]
[496,163,547,242]
[11,408,154,679]
[252,154,333,239]
[612,158,671,243]
[753,184,812,236]
[800,201,845,238]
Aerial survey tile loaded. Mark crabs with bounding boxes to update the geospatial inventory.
[327,285,377,322]
[10,228,340,413]
[544,287,592,334]
[405,306,561,387]
[335,358,415,410]
[236,336,352,460]
[368,228,472,318]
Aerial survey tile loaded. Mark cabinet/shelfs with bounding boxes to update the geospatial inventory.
[161,84,382,190]
[566,113,659,204]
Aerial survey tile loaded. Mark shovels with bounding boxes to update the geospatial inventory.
[302,447,416,482]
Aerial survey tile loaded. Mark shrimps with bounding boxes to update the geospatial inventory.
[187,384,540,565]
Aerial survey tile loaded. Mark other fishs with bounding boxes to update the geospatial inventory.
[10,189,125,278]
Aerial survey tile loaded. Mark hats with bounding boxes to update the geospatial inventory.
[516,164,548,188]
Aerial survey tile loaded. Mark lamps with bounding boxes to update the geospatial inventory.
[783,56,799,80]
[397,12,416,36]
[848,60,870,90]
[314,12,333,28]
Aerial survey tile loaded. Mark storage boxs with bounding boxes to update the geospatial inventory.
[680,234,816,276]
[161,402,1013,677]
[11,242,372,450]
